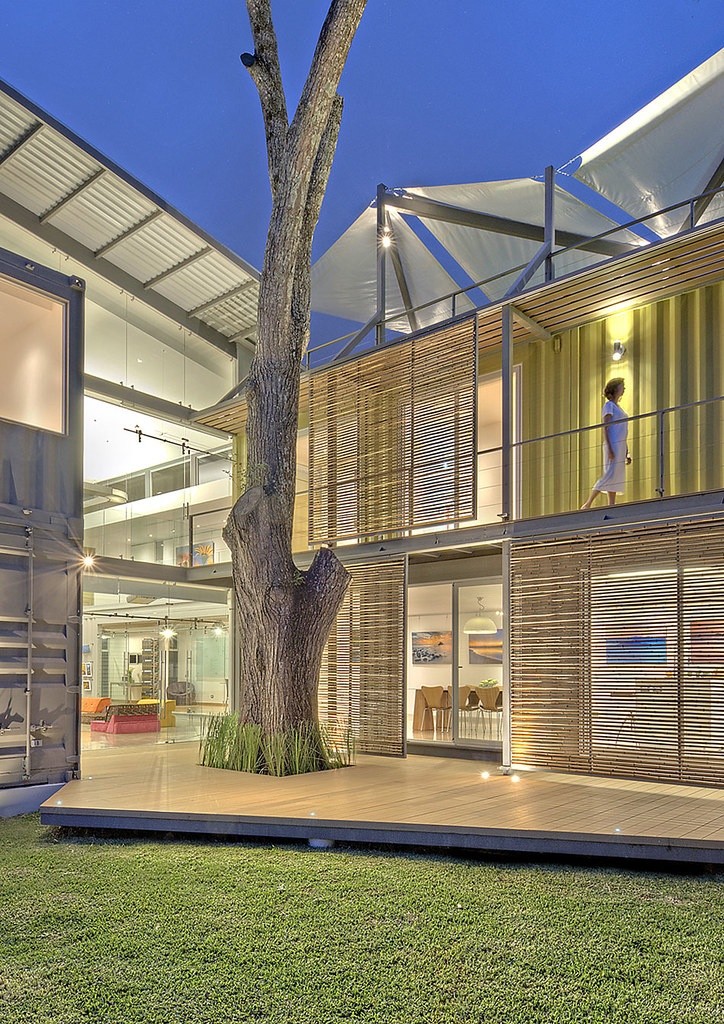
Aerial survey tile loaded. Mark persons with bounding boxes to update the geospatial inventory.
[579,375,633,509]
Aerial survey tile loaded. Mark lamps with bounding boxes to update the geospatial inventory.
[463,596,497,635]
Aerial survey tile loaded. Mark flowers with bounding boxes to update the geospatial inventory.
[479,678,498,689]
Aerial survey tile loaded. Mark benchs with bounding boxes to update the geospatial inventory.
[80,696,176,737]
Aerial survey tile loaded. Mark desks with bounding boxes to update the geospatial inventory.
[111,681,152,701]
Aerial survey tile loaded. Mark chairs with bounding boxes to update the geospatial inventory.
[166,681,196,704]
[414,684,503,743]
